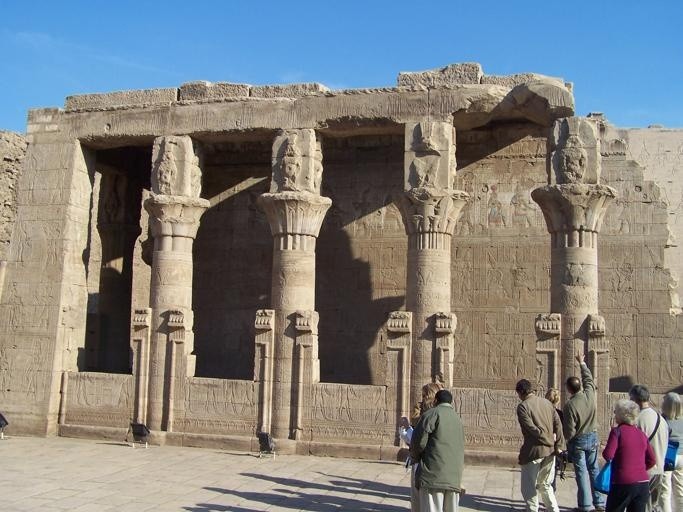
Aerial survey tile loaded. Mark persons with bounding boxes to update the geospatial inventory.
[544,387,565,494]
[398,383,439,512]
[600,399,657,512]
[627,384,670,512]
[661,390,683,512]
[562,350,606,512]
[406,389,466,512]
[516,378,565,512]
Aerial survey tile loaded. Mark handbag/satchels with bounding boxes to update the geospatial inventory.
[664,441,679,471]
[593,460,612,494]
